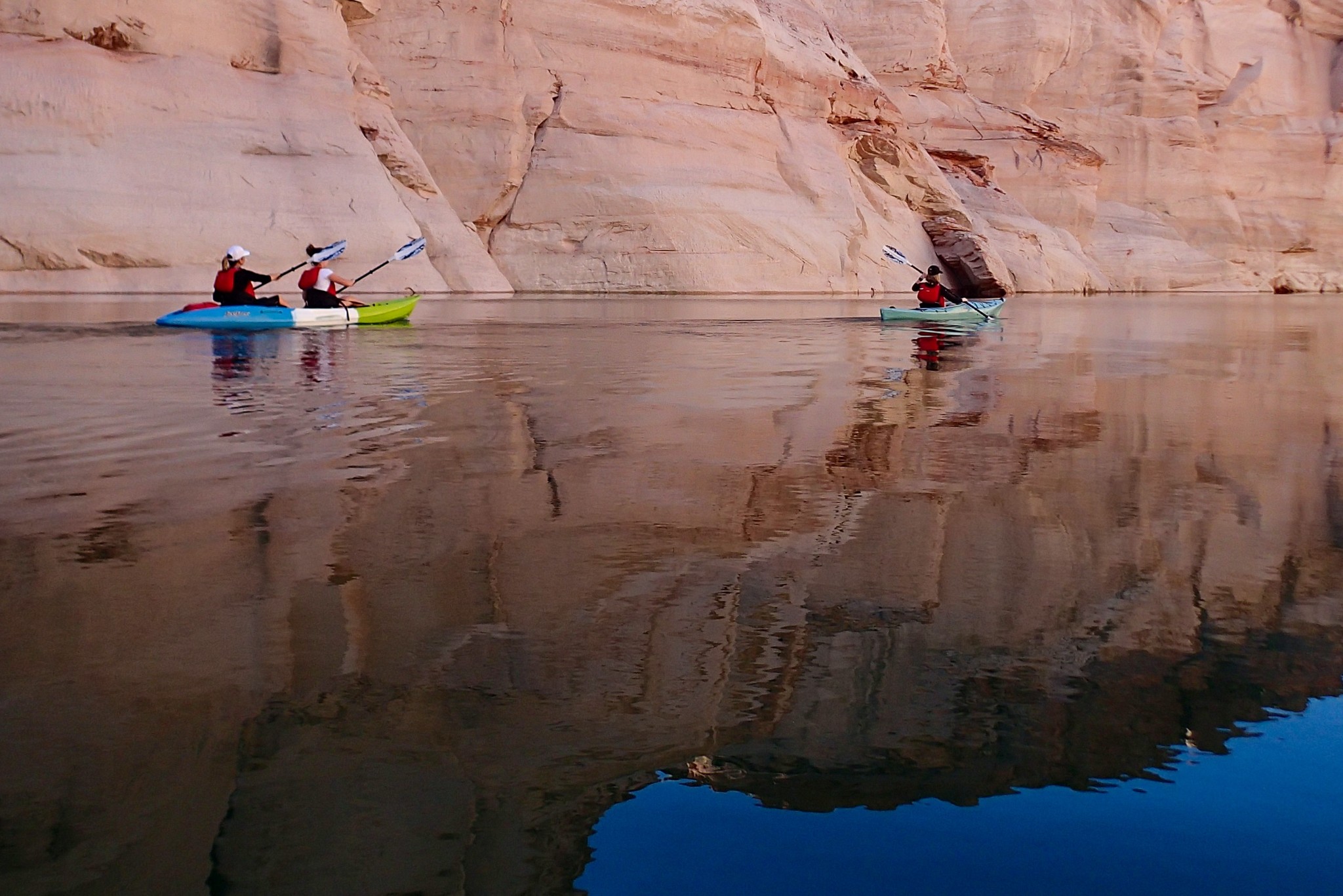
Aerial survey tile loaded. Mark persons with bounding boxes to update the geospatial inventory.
[212,245,293,308]
[911,265,968,308]
[298,245,369,309]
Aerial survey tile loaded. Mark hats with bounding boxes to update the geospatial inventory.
[928,265,943,275]
[227,246,250,261]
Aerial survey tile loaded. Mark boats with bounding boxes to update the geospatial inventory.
[877,295,1010,323]
[156,290,424,329]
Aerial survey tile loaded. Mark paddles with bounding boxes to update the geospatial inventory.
[335,236,427,296]
[882,244,994,321]
[252,238,348,291]
